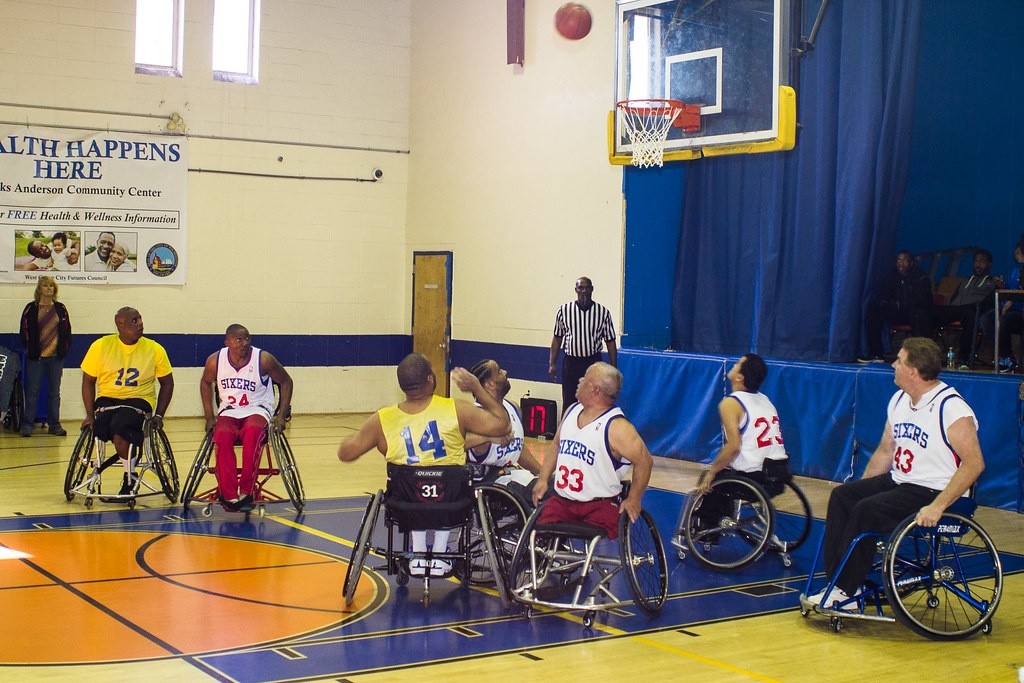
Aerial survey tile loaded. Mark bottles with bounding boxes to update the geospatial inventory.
[947,346,956,370]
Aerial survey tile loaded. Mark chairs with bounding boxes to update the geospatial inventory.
[883,276,1005,367]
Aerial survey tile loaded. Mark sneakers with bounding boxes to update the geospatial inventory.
[409,558,426,574]
[20,427,32,437]
[48,424,66,436]
[429,558,452,576]
[808,586,858,610]
[854,588,861,596]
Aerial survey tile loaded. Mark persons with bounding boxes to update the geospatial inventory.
[200,324,294,512]
[857,250,925,363]
[928,250,996,370]
[687,354,789,546]
[15,233,80,270]
[549,277,617,420]
[86,232,136,271]
[80,307,174,495]
[979,241,1024,374]
[533,361,653,539]
[20,276,71,437]
[465,360,555,509]
[337,354,512,578]
[807,336,986,610]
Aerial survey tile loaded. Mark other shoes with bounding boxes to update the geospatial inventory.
[712,534,720,545]
[958,360,970,370]
[857,353,885,363]
[226,498,238,504]
[999,356,1019,373]
[119,472,140,495]
[238,494,252,511]
[699,535,712,544]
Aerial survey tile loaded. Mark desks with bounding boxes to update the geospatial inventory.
[995,289,1024,377]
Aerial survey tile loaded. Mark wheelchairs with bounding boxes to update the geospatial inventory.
[179,415,307,514]
[64,416,181,510]
[798,515,1005,641]
[671,475,812,571]
[341,480,669,627]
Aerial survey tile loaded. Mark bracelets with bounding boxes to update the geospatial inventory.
[155,414,163,419]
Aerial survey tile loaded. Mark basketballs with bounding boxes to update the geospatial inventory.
[555,3,593,41]
